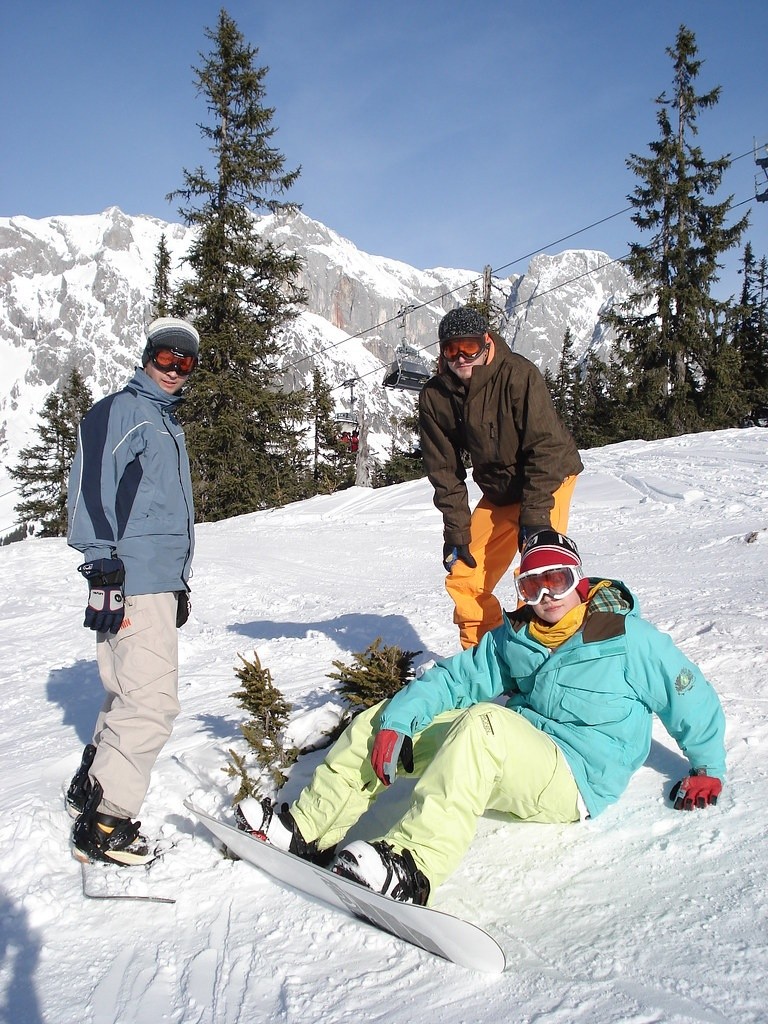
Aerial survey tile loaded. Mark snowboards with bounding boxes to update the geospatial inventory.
[57,772,177,905]
[181,797,509,976]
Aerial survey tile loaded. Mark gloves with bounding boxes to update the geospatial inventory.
[443,544,477,572]
[518,525,555,552]
[371,730,414,786]
[77,558,126,634]
[669,776,722,810]
[176,593,191,628]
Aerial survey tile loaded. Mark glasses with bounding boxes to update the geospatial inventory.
[149,344,198,373]
[440,334,486,361]
[513,564,586,605]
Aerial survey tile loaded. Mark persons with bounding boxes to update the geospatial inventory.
[64,315,200,869]
[233,529,728,909]
[418,304,584,653]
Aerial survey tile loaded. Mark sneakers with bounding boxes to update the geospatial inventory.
[72,781,161,867]
[63,745,96,818]
[336,839,431,907]
[233,798,336,868]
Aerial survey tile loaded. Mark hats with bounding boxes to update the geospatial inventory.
[520,530,589,599]
[141,317,199,367]
[438,307,486,337]
